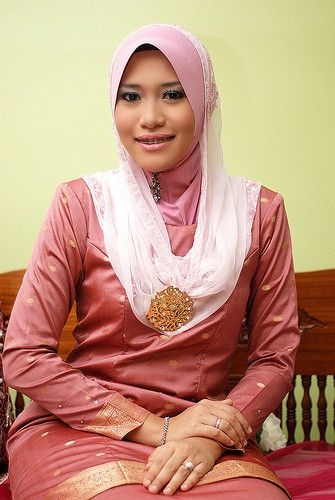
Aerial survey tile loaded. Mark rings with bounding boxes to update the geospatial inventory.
[182,461,194,472]
[215,417,221,428]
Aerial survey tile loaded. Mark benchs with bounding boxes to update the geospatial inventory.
[0,268,335,500]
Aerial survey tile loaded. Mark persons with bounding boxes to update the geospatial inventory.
[1,24,299,500]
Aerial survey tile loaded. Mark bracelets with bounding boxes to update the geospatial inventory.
[158,416,171,446]
